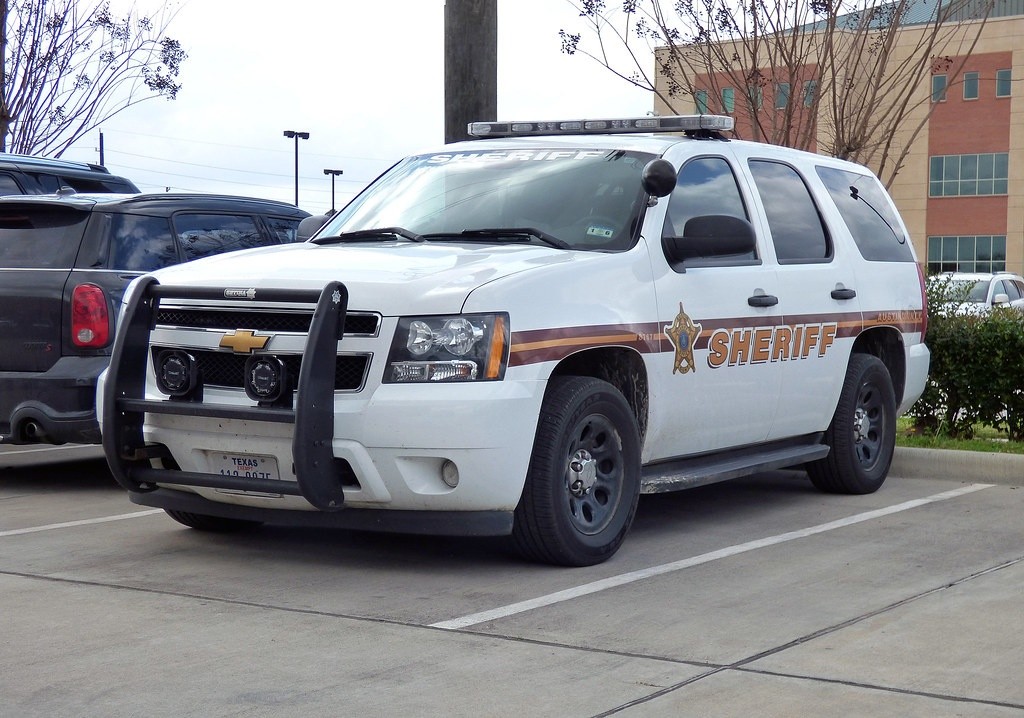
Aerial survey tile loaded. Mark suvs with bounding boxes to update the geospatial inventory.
[0,188,326,472]
[0,153,144,204]
[96,114,933,572]
[922,270,1024,325]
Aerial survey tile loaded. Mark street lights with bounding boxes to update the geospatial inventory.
[322,167,343,217]
[283,129,310,207]
